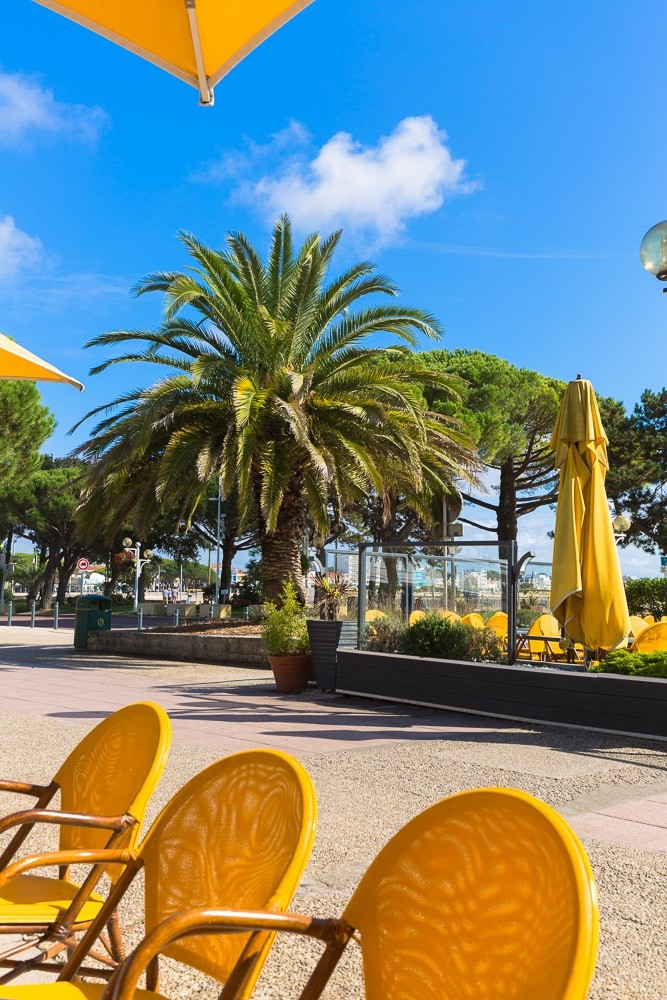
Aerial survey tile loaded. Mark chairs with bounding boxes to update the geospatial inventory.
[0,702,595,1000]
[365,610,667,666]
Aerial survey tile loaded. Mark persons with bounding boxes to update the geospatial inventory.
[123,591,135,605]
[162,587,178,604]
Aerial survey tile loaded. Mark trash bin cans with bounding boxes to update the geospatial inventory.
[73,594,113,650]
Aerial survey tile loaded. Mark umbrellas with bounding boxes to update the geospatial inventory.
[0,331,83,392]
[33,0,313,106]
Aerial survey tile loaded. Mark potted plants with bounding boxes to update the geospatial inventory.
[259,571,354,694]
